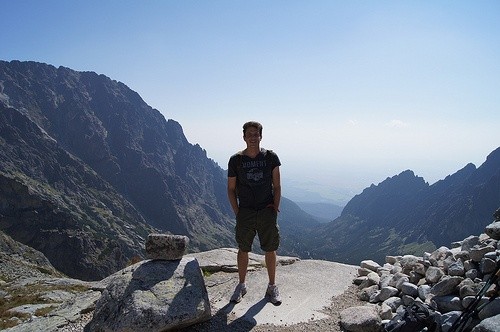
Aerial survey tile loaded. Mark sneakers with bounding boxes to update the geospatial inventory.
[230,284,248,303]
[265,285,282,304]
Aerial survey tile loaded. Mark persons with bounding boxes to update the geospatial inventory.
[227,121,283,306]
